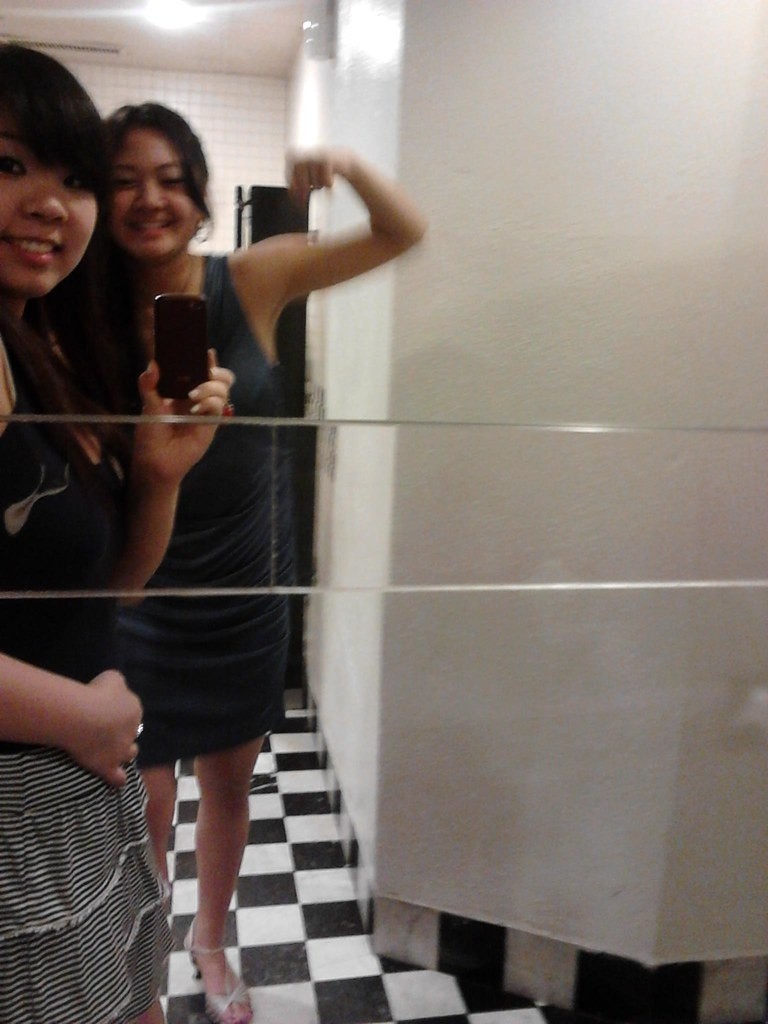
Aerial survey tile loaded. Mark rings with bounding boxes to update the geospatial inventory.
[136,723,144,738]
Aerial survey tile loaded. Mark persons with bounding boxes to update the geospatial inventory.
[0,42,237,1023]
[100,103,426,1023]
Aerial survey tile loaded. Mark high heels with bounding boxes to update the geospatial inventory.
[185,915,253,1024]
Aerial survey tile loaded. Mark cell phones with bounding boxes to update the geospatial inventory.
[154,292,212,400]
